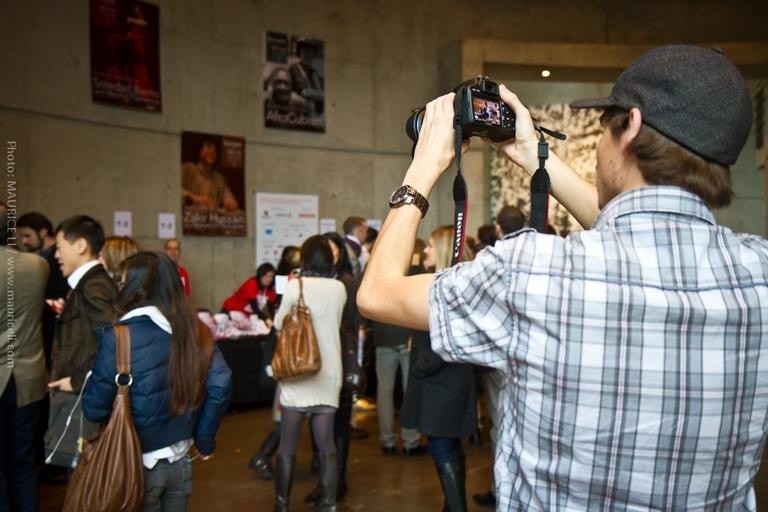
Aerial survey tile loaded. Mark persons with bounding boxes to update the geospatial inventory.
[289,35,324,113]
[354,42,768,511]
[263,68,312,123]
[179,138,239,214]
[0,201,529,512]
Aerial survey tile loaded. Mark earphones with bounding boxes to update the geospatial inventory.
[71,460,78,468]
[45,454,52,464]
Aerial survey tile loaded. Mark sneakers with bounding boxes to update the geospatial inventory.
[474,491,495,507]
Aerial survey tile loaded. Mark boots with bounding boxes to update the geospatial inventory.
[249,432,348,512]
[438,452,468,512]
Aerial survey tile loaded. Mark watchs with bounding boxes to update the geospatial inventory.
[389,183,430,220]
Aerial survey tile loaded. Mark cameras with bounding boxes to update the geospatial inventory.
[406,77,515,160]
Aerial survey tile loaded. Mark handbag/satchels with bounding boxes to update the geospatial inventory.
[271,305,321,381]
[64,387,144,511]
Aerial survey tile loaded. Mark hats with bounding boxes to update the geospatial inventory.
[571,45,753,164]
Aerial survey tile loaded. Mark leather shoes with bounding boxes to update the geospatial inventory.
[351,427,427,457]
